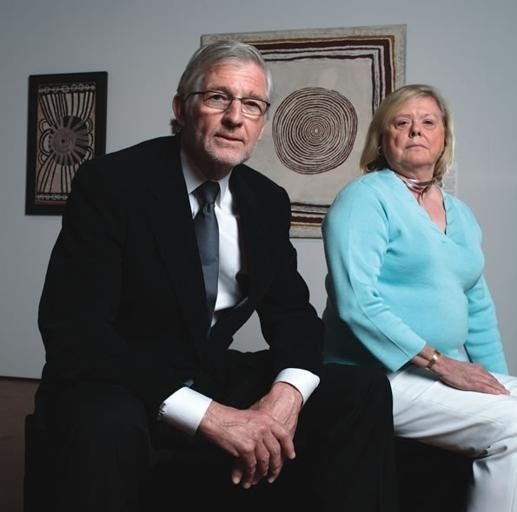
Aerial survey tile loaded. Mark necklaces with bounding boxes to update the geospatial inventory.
[401,175,437,200]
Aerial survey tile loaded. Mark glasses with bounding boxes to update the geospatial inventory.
[183,89,271,116]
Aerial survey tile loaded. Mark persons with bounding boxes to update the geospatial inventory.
[26,39,397,511]
[321,83,516,512]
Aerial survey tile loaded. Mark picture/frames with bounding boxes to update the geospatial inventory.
[199,22,408,238]
[25,69,108,216]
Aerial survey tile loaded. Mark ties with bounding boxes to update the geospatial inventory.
[191,180,219,327]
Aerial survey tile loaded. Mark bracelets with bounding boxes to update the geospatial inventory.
[424,350,440,372]
